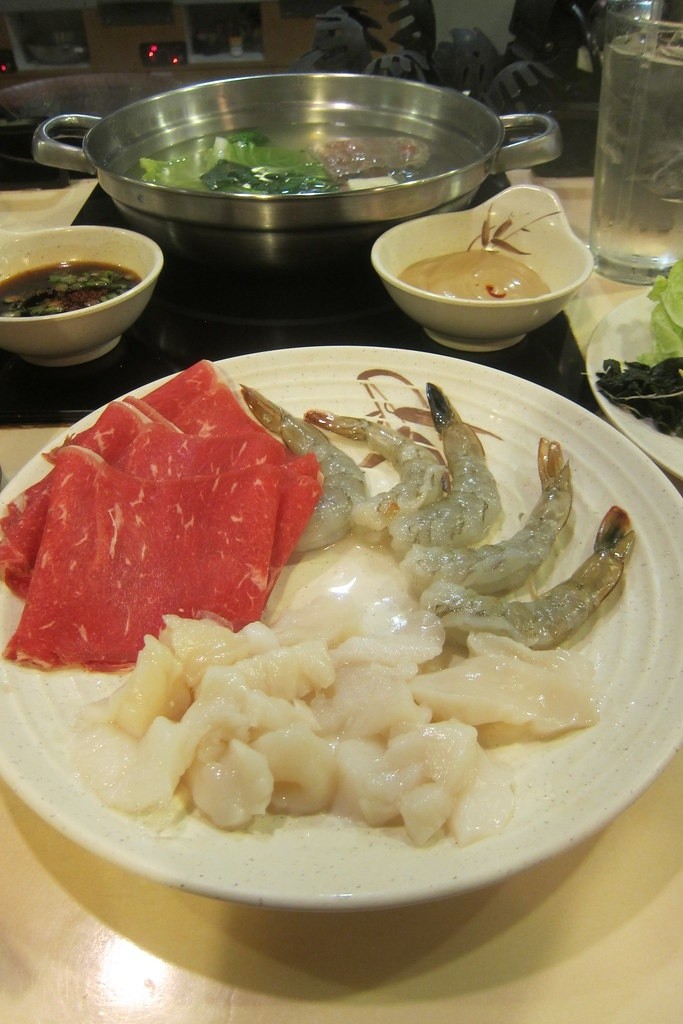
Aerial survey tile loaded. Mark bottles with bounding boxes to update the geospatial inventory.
[230,29,243,58]
[590,0,683,287]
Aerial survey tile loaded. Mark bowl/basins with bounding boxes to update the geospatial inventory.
[371,183,595,352]
[0,225,164,366]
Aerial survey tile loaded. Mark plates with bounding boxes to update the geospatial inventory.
[0,344,682,910]
[587,290,683,479]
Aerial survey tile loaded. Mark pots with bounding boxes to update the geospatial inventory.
[110,187,482,327]
[32,72,563,230]
[26,29,88,66]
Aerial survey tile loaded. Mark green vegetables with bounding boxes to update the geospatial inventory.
[594,261,683,438]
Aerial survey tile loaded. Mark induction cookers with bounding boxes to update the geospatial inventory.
[0,171,598,430]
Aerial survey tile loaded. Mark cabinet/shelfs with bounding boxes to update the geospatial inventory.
[0,0,403,77]
